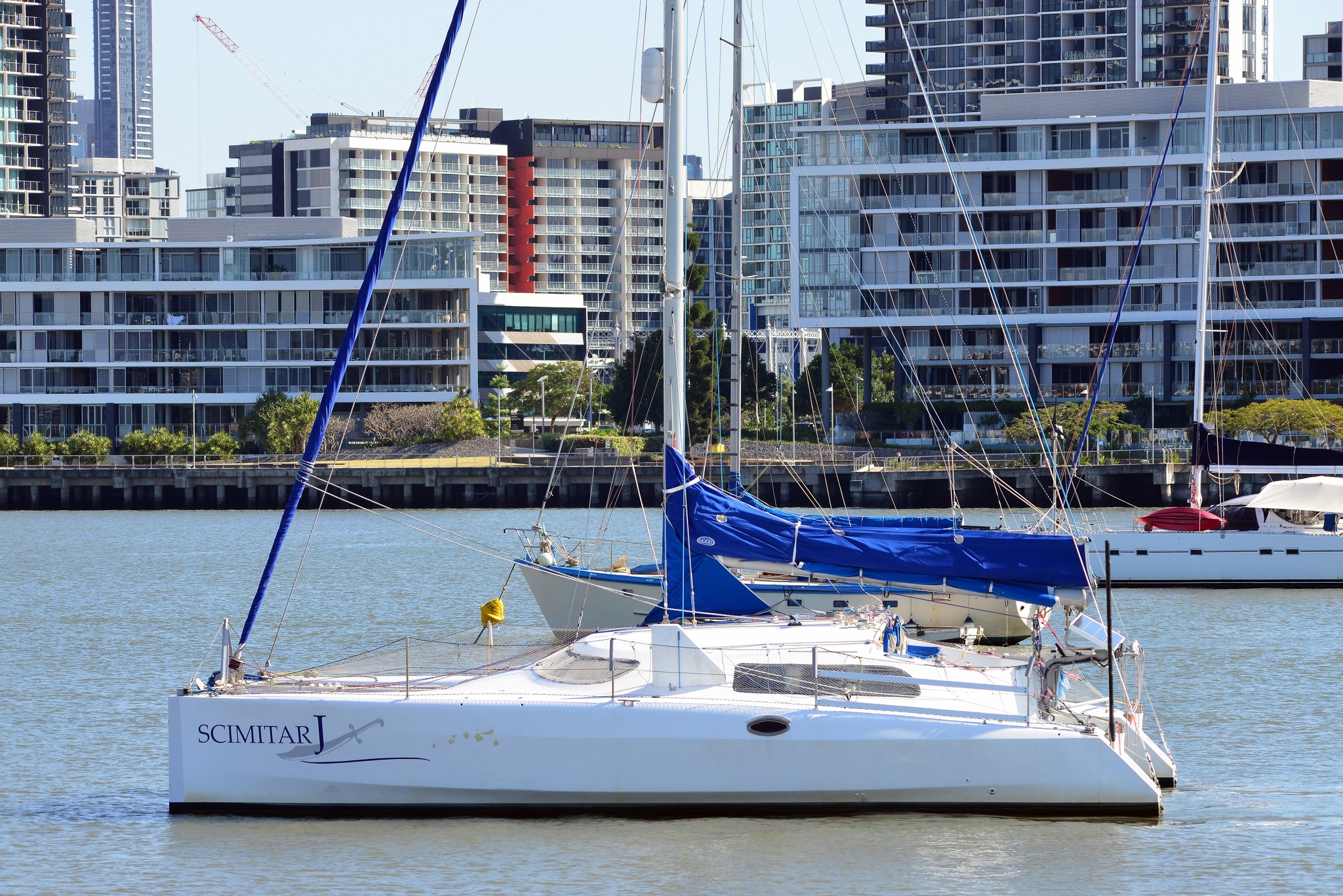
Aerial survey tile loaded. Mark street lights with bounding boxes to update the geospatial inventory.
[791,385,797,466]
[584,372,596,431]
[537,375,548,435]
[826,383,833,461]
[192,389,198,468]
[856,373,864,414]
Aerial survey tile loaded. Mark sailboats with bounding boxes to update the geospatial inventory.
[1057,0,1343,590]
[166,0,1182,830]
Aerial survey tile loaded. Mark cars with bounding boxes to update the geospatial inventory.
[597,425,617,432]
[638,428,655,433]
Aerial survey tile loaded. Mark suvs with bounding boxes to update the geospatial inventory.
[795,423,815,431]
